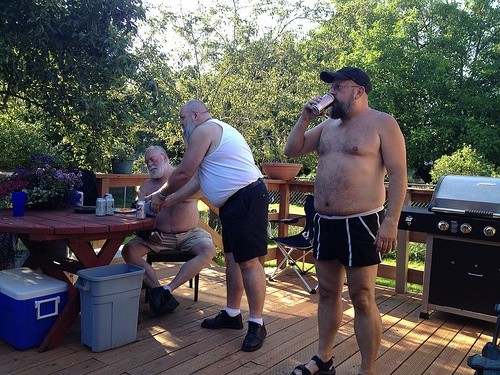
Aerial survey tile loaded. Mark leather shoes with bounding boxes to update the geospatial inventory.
[201,310,243,330]
[241,320,267,351]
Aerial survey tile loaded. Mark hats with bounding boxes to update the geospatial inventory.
[320,67,372,95]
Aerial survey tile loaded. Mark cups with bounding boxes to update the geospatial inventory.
[12,192,26,216]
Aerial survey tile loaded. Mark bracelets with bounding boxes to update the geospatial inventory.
[157,191,166,200]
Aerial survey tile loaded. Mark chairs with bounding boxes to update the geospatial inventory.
[268,195,318,294]
[132,200,199,304]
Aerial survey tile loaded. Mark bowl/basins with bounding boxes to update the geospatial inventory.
[260,162,303,180]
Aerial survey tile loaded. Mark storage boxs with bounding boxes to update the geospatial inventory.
[0,267,68,350]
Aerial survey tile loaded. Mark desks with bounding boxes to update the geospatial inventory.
[0,209,156,353]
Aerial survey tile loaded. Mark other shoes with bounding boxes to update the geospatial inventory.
[148,286,180,321]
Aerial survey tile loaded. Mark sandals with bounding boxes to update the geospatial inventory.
[290,355,336,375]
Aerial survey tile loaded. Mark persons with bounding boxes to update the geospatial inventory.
[284,67,408,375]
[145,99,270,351]
[121,146,216,315]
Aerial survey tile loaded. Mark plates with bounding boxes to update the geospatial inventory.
[113,207,136,213]
[74,206,95,213]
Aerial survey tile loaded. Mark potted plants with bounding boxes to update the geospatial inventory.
[259,145,330,186]
[106,131,141,174]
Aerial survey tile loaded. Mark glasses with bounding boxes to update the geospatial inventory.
[328,84,362,92]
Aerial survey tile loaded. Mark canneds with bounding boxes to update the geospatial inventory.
[136,200,146,219]
[76,192,83,206]
[312,92,335,115]
[96,193,114,216]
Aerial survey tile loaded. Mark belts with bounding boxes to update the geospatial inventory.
[223,179,262,207]
